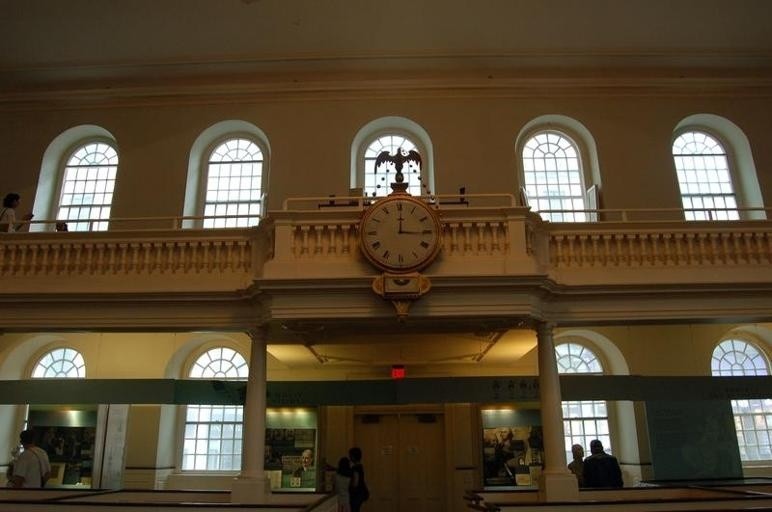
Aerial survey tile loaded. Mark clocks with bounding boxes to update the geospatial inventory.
[357,182,443,323]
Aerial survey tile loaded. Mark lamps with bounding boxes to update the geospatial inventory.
[391,364,406,380]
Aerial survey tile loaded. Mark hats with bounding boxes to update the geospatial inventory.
[349,448,361,453]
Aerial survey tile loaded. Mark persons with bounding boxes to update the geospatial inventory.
[293,448,314,478]
[567,444,585,489]
[330,456,354,511]
[581,439,624,489]
[264,444,283,470]
[1,192,21,233]
[495,426,515,461]
[348,447,367,512]
[10,429,53,488]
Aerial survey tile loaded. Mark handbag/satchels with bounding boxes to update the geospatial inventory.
[357,485,369,500]
